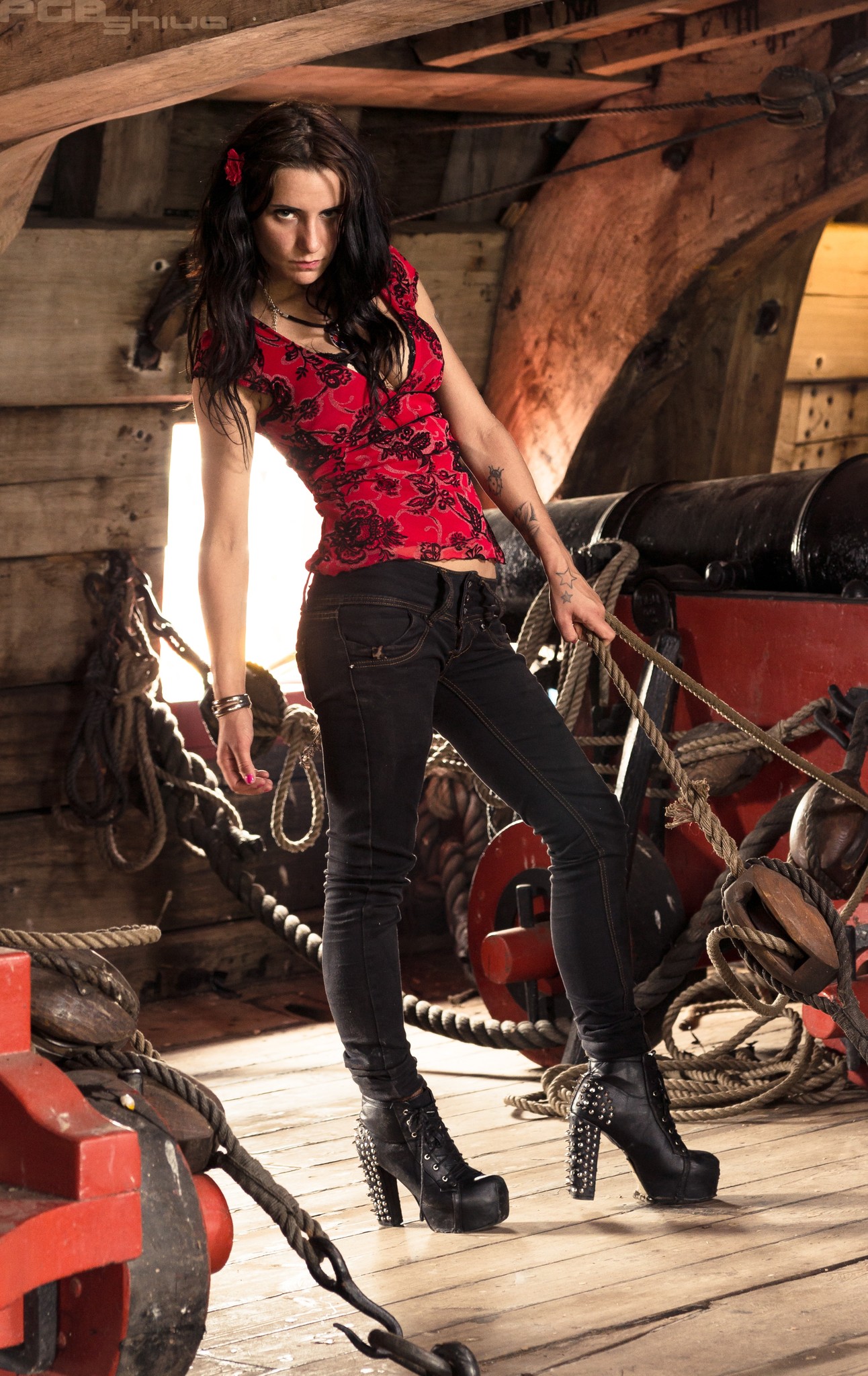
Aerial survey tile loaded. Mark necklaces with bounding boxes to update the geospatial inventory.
[253,280,349,352]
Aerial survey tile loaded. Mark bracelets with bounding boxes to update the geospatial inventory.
[212,694,249,711]
[212,699,252,718]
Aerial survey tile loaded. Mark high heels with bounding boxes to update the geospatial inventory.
[353,1088,510,1233]
[570,1055,721,1204]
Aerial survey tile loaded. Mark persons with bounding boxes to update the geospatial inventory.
[155,102,721,1230]
